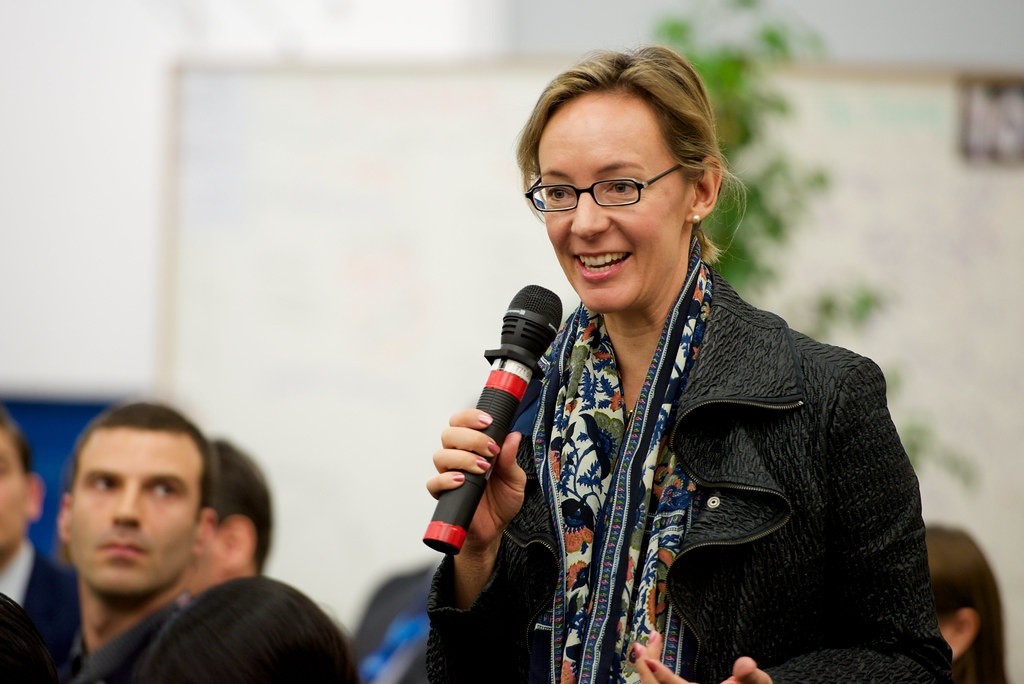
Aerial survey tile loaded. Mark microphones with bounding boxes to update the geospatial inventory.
[422,284,563,557]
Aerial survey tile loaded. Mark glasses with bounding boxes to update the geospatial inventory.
[524,157,702,212]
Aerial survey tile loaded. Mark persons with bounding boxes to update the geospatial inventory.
[926,524,1011,684]
[422,47,955,684]
[0,402,363,684]
